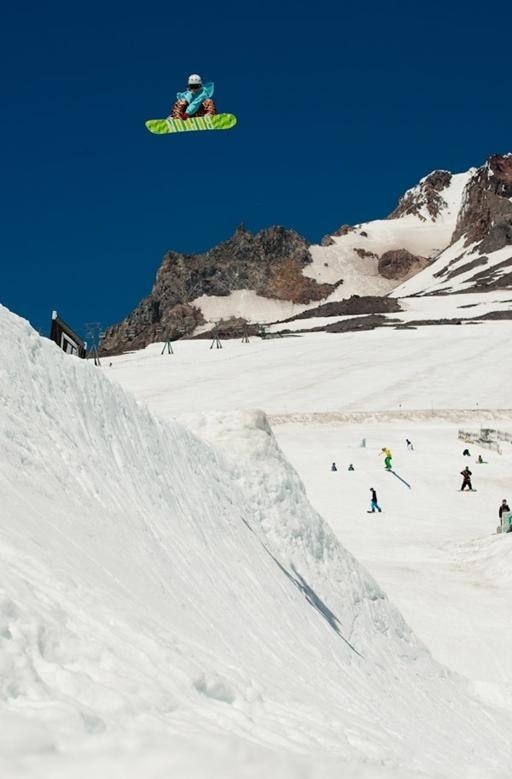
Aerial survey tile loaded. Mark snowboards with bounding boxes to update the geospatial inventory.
[145,114,236,134]
[456,488,477,492]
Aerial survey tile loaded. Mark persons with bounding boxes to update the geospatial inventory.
[459,466,474,492]
[406,439,414,451]
[331,462,337,471]
[369,487,382,513]
[462,449,470,457]
[347,463,354,470]
[377,447,392,472]
[499,499,510,527]
[478,456,488,464]
[169,70,218,120]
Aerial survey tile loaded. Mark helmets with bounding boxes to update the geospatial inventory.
[188,74,201,84]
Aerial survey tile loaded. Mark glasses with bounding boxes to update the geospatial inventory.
[189,84,200,89]
